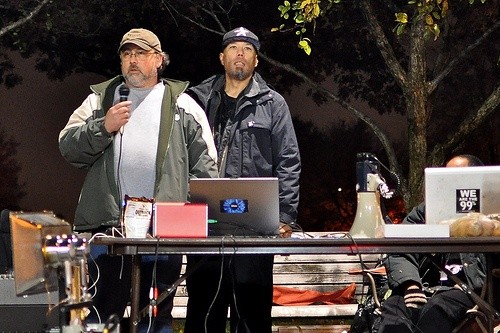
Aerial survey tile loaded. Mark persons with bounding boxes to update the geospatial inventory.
[0,132,35,276]
[372,155,488,333]
[59,29,219,333]
[183,27,300,333]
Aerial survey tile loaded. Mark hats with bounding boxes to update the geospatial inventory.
[117,28,161,52]
[222,27,259,54]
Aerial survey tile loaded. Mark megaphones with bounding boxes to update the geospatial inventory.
[349,153,385,239]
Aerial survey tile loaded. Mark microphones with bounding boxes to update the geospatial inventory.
[118,87,129,134]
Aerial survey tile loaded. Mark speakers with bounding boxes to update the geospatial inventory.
[10,210,71,294]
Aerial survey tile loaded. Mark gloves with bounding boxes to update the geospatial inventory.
[404,289,427,318]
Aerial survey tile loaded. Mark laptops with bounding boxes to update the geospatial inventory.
[424,166,500,224]
[190,176,281,235]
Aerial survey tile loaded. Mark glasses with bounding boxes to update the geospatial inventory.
[122,51,158,57]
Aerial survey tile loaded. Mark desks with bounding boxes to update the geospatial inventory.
[91,237,500,333]
